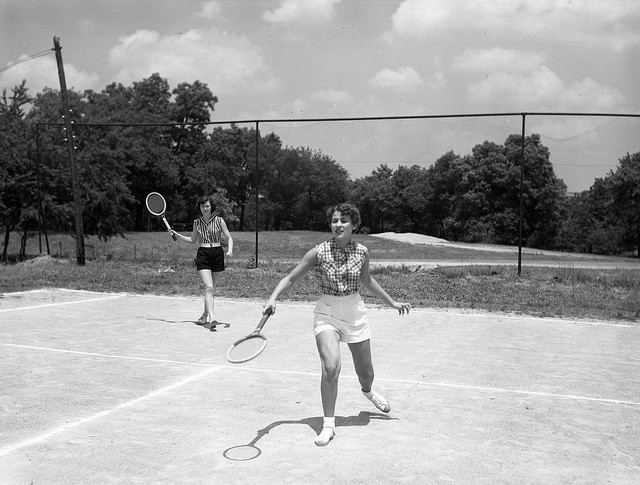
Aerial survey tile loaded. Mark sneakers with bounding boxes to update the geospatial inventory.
[210,320,216,330]
[315,426,336,446]
[197,315,207,324]
[366,393,390,413]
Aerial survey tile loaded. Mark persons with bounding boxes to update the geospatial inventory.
[168,196,233,327]
[262,204,411,445]
